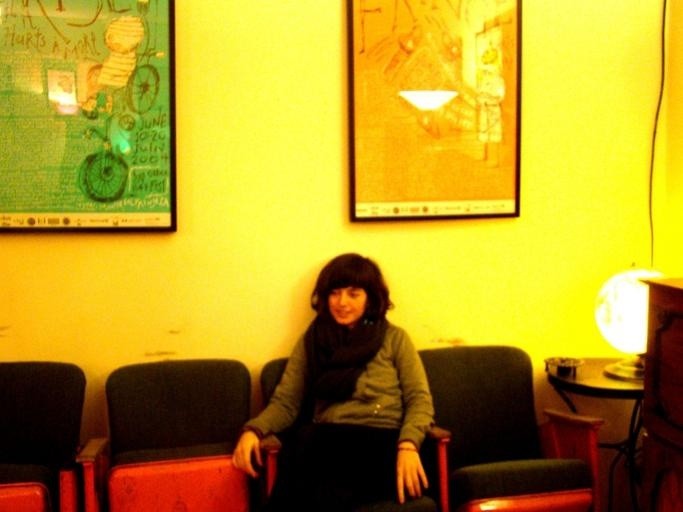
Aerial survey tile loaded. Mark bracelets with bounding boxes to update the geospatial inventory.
[397,447,419,452]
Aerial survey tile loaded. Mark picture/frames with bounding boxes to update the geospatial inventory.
[0,0,177,234]
[346,1,523,224]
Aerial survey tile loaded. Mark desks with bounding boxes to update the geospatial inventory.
[546,356,644,507]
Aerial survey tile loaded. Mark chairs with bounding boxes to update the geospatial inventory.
[78,355,258,510]
[2,357,80,510]
[258,357,290,494]
[416,343,607,510]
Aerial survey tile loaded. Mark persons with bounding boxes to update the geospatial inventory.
[230,253,435,512]
